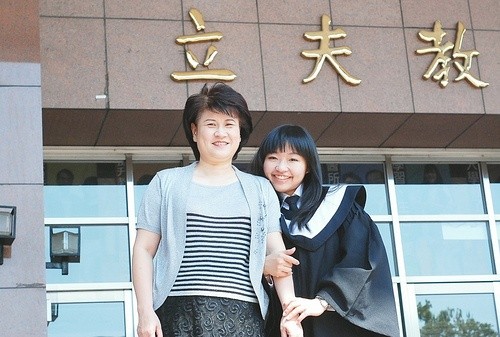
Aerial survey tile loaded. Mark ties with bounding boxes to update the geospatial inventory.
[286,196,299,213]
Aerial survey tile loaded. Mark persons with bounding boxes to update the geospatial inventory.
[251,126,398,336]
[131,81,304,337]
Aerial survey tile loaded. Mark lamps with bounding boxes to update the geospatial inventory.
[0,205,17,265]
[45,223,81,275]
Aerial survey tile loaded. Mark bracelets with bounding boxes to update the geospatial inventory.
[315,296,329,309]
[265,275,270,278]
